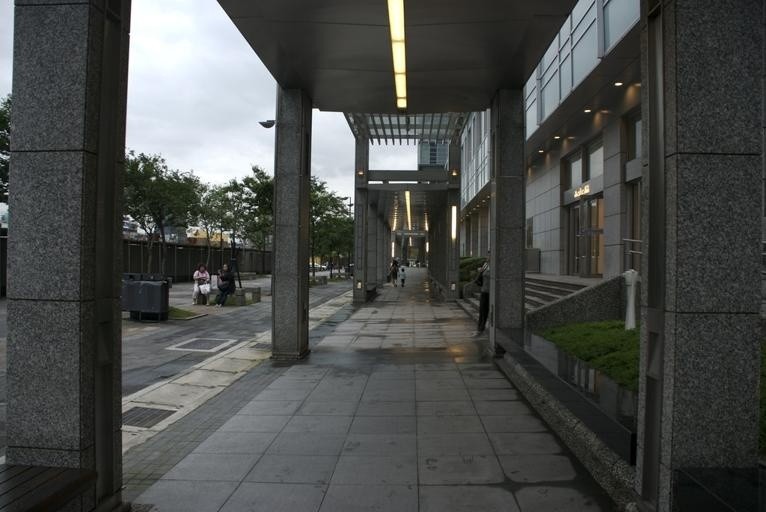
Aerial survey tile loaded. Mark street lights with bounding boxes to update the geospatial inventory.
[339,196,355,216]
[257,120,277,129]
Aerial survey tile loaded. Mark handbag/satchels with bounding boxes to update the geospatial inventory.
[199,283,211,294]
[474,272,483,287]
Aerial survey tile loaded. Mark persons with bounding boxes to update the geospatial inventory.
[390,260,399,287]
[398,268,407,288]
[192,264,211,306]
[214,263,236,308]
[473,250,491,338]
